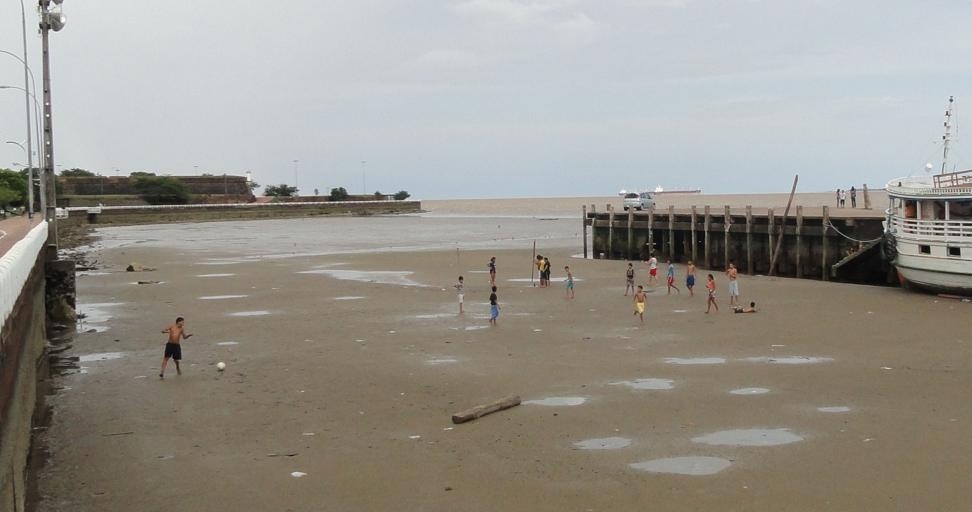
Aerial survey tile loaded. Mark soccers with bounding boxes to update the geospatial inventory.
[217,362,226,370]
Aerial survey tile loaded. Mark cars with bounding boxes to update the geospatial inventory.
[623,192,656,211]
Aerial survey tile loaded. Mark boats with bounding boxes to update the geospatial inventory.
[879,94,972,295]
[654,185,704,194]
[618,190,627,195]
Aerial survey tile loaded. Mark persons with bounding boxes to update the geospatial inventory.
[488,256,496,285]
[489,286,501,326]
[624,263,634,296]
[730,301,756,313]
[705,274,719,313]
[645,253,657,284]
[686,261,695,296]
[666,259,680,294]
[454,276,465,313]
[159,317,192,377]
[633,285,647,321]
[536,255,545,288]
[726,263,738,304]
[544,257,551,287]
[564,266,575,299]
[836,186,856,207]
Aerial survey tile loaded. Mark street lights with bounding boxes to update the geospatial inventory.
[293,159,299,195]
[0,85,46,220]
[360,160,367,194]
[36,0,67,257]
